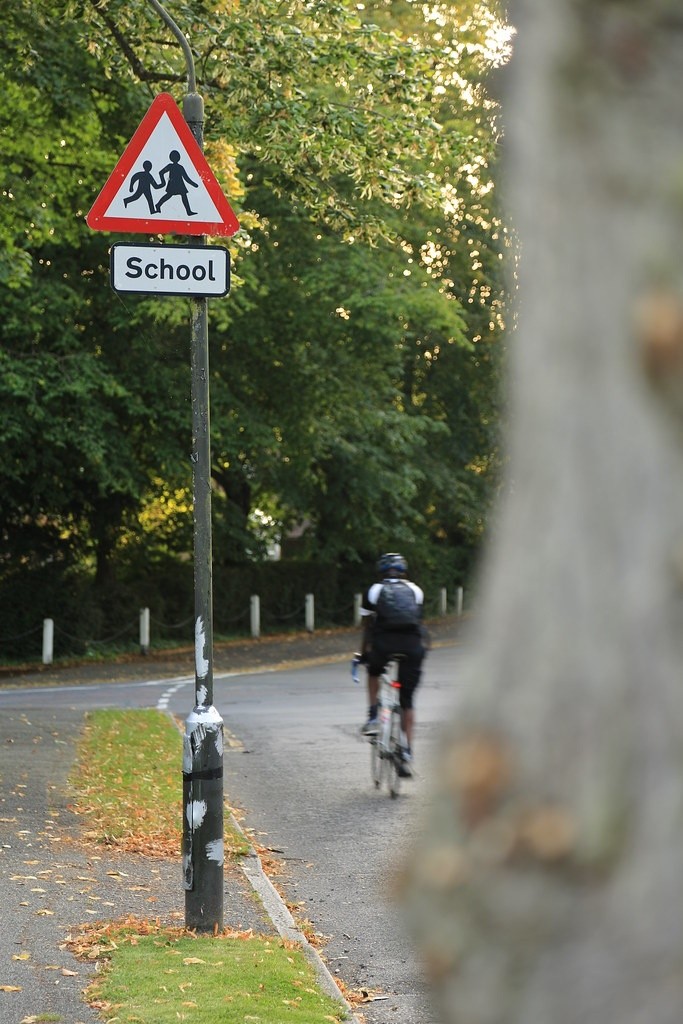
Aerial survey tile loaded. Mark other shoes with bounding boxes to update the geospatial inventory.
[394,743,412,762]
[359,721,380,735]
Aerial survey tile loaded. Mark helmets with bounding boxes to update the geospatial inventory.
[376,552,408,573]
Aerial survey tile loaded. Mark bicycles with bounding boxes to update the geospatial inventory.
[346,646,433,801]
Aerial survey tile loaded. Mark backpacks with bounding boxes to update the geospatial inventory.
[376,581,419,634]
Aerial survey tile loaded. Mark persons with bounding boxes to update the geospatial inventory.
[361,552,425,778]
